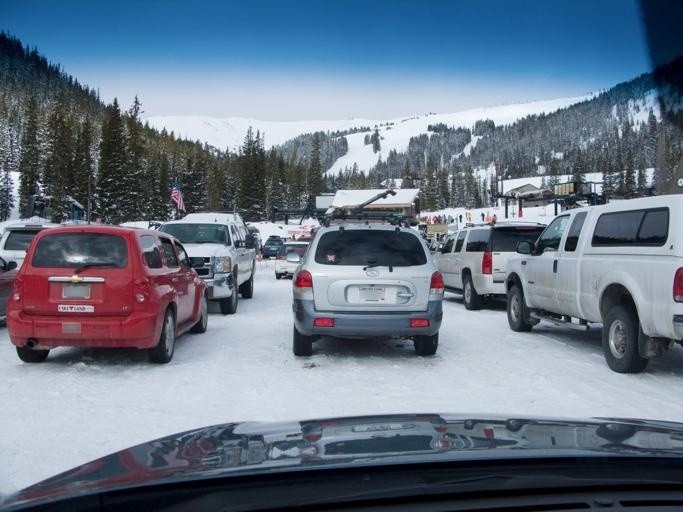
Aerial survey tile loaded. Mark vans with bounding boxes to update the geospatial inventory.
[497,192,682,377]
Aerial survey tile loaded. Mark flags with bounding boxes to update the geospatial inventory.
[169,177,186,212]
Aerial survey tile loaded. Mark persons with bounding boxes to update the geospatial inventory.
[419,211,496,225]
